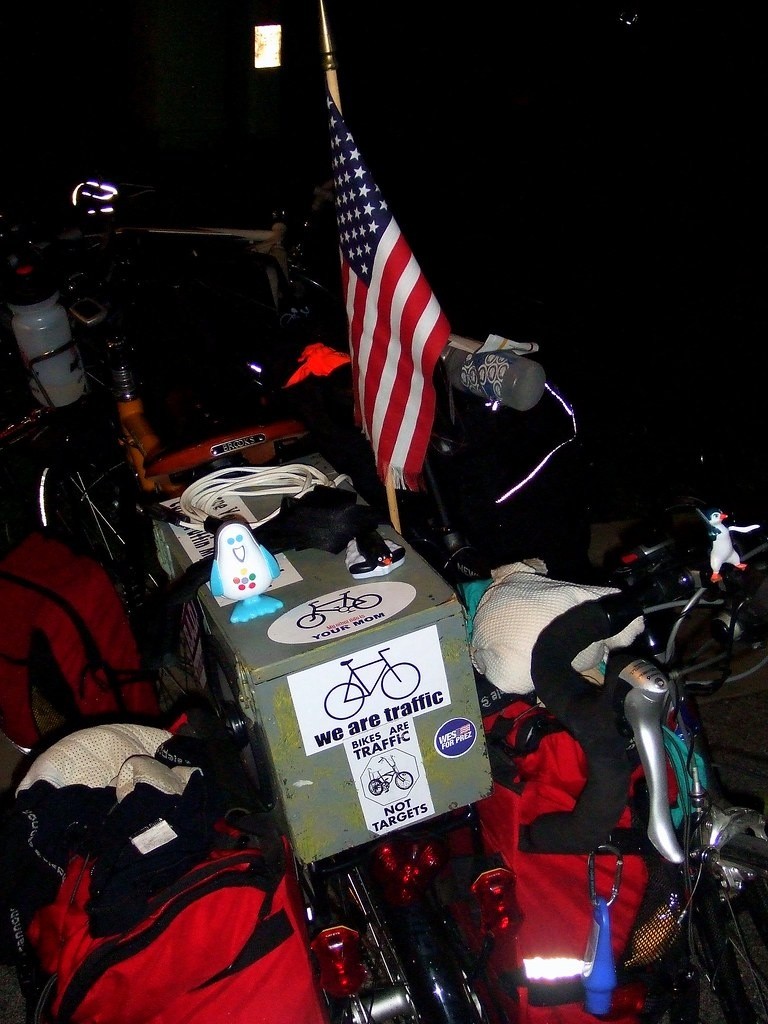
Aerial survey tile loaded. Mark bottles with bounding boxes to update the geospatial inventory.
[4,260,86,407]
[441,336,546,412]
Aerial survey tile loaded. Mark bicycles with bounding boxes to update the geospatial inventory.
[0,177,768,1024]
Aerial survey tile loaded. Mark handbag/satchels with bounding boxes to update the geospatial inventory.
[0,537,161,758]
[22,797,330,1024]
[445,691,691,1024]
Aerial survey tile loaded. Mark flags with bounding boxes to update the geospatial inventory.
[320,91,452,495]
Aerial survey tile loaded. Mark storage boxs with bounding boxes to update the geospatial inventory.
[154,452,496,869]
